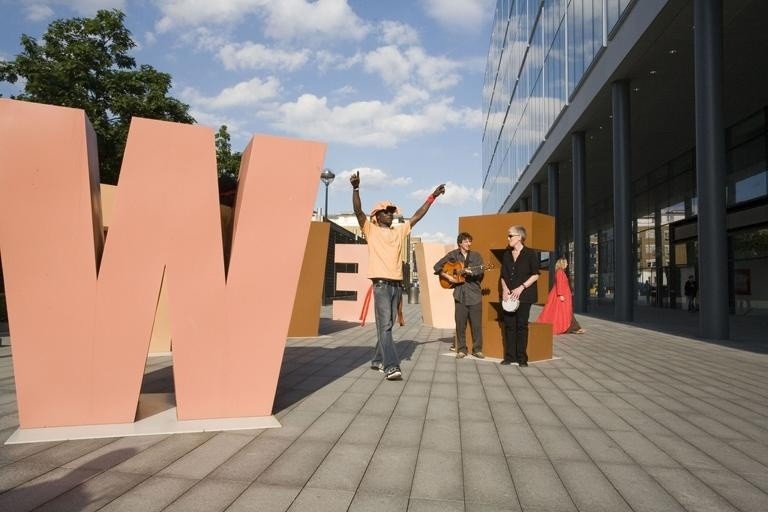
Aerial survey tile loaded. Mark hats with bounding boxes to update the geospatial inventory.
[368,201,399,216]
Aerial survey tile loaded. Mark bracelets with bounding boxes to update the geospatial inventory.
[521,283,526,289]
[427,194,436,205]
[353,187,359,191]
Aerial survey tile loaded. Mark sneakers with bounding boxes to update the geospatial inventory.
[472,350,484,358]
[371,362,401,380]
[518,358,528,367]
[457,349,466,358]
[502,359,516,365]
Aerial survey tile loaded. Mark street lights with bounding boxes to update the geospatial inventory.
[321,168,335,218]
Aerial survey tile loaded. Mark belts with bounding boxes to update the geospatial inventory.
[373,279,400,286]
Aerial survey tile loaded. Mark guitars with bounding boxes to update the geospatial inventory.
[439,261,494,288]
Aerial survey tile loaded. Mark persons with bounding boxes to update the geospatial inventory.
[433,232,485,359]
[500,225,541,367]
[685,275,698,313]
[642,280,652,304]
[350,170,445,380]
[535,257,584,335]
